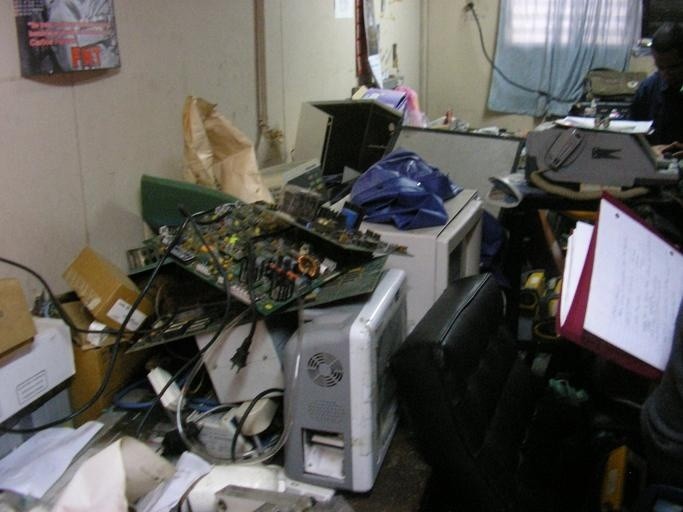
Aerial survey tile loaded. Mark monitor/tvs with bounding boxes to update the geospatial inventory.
[283,267,408,492]
[642,0,683,37]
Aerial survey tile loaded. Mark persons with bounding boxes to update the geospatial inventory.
[622,22,682,160]
[639,296,683,468]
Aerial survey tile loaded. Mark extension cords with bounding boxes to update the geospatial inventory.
[146,367,186,414]
